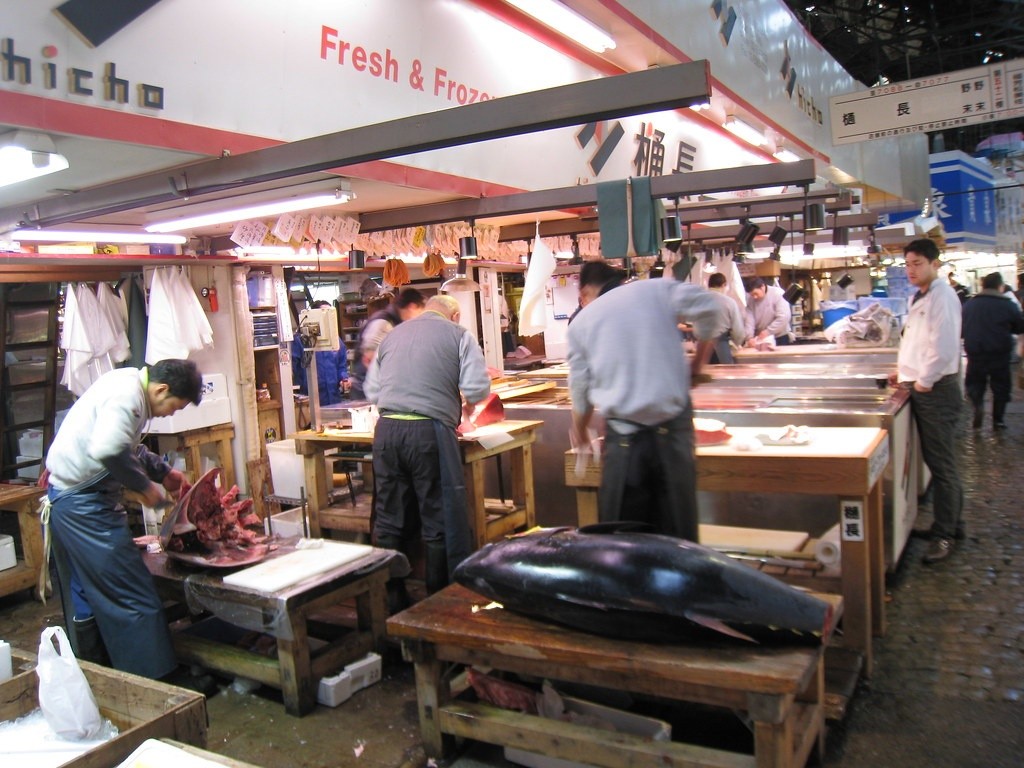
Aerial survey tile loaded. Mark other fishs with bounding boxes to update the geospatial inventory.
[160,465,283,573]
[454,521,838,654]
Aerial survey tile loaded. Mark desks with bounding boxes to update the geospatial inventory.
[1,423,888,768]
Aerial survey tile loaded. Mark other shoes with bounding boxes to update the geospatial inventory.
[972,413,983,428]
[991,421,1009,430]
[922,526,966,541]
[923,537,950,560]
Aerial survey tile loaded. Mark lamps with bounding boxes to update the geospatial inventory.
[659,195,681,243]
[349,243,365,272]
[0,129,70,188]
[458,219,478,260]
[734,205,882,304]
[143,190,357,233]
[11,229,190,246]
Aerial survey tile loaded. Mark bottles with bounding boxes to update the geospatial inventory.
[258,383,272,401]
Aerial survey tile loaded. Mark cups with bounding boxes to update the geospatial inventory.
[342,379,350,393]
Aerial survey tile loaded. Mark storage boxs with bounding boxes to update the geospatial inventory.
[264,436,339,499]
[501,691,672,768]
[304,634,384,709]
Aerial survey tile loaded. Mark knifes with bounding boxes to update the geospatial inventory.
[157,501,175,510]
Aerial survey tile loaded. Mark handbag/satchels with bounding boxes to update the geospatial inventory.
[34,626,102,741]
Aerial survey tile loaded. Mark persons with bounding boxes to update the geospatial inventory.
[363,294,491,610]
[693,272,745,366]
[961,272,1024,430]
[888,239,968,561]
[947,272,972,304]
[291,300,351,406]
[1004,280,1024,364]
[340,287,425,453]
[566,260,732,544]
[745,278,792,345]
[35,358,202,684]
